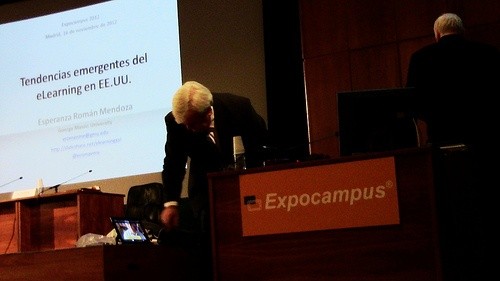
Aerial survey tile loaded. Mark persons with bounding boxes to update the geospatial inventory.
[406,12,500,281]
[161,80,267,281]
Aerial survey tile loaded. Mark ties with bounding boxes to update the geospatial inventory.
[206,126,217,145]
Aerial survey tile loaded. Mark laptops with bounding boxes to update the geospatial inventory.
[110,216,154,245]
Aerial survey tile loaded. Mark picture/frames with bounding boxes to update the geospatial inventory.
[110,217,151,245]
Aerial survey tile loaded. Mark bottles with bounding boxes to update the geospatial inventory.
[235,153,246,170]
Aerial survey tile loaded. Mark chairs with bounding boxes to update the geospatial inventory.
[123,182,163,232]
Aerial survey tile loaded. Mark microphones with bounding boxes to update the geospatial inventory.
[49,170,92,193]
[0,177,23,188]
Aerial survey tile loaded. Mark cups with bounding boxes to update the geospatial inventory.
[233,136,245,155]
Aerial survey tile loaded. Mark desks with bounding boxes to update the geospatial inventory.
[0,191,128,254]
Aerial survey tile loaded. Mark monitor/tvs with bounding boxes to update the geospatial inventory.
[337,87,418,156]
